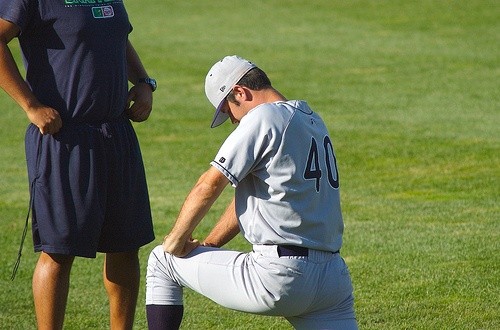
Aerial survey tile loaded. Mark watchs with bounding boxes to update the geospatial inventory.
[142,78,157,91]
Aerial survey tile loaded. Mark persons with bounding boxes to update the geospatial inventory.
[0,0,158,330]
[145,55,358,330]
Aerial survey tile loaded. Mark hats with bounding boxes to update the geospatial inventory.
[204,55,256,128]
